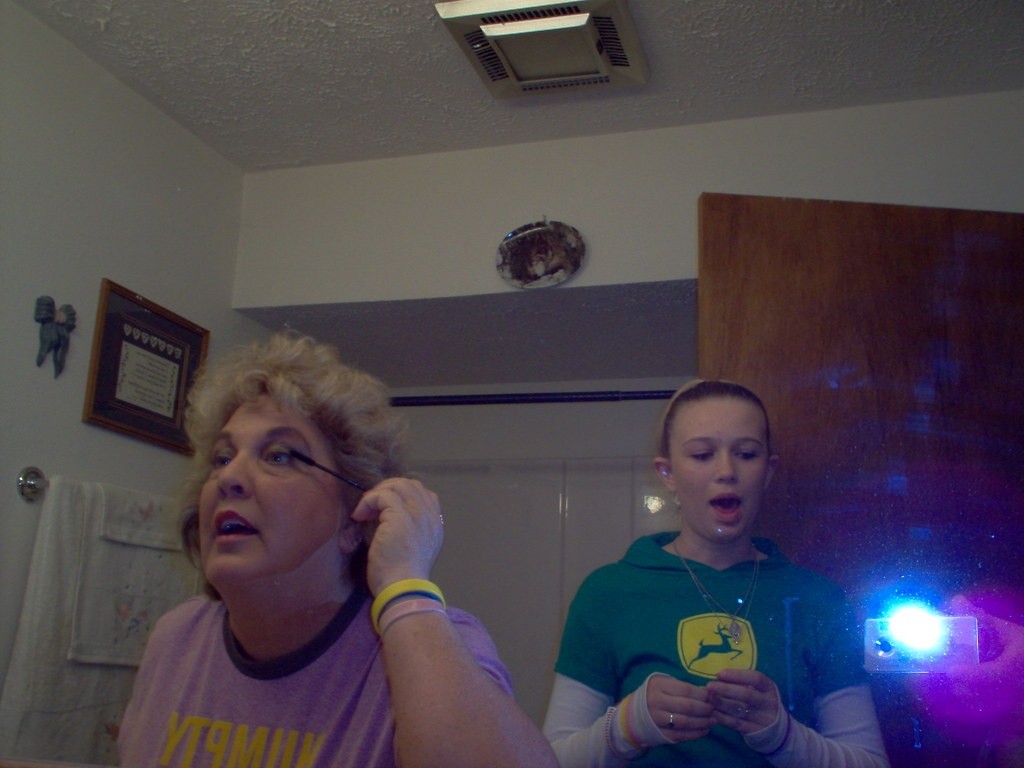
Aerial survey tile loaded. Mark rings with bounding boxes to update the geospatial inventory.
[669,713,676,725]
[735,703,751,719]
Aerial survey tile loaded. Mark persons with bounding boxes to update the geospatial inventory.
[902,598,1024,741]
[116,325,561,768]
[538,376,890,768]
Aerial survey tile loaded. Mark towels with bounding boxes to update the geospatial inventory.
[4,475,206,765]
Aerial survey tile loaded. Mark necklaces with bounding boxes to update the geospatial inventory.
[670,531,768,646]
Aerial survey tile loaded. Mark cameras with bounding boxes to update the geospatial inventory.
[863,616,979,672]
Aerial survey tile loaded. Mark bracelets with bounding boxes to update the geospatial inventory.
[370,574,452,634]
[602,703,650,763]
[745,699,792,758]
[620,692,643,748]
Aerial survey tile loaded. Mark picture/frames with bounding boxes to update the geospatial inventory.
[82,277,209,459]
